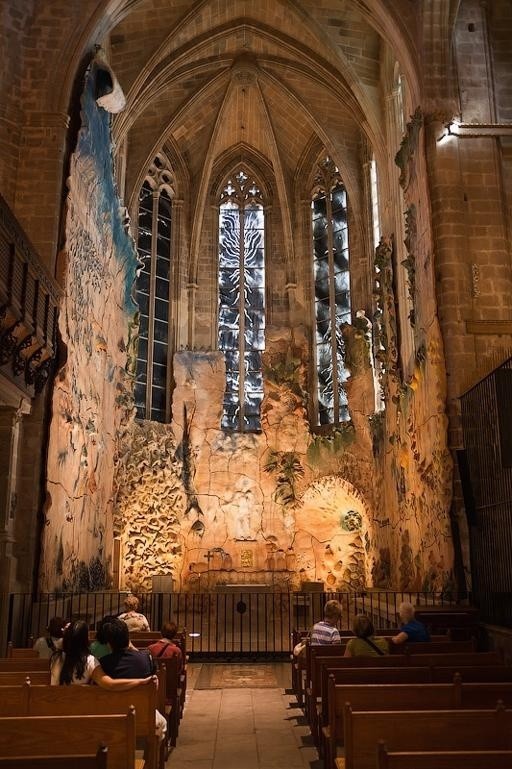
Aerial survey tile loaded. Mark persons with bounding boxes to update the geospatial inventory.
[309,600,432,657]
[32,596,182,742]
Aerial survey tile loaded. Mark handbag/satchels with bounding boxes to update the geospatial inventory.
[293,639,306,658]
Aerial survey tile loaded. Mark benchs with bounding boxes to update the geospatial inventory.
[73,589,134,629]
[1,628,187,769]
[288,626,512,767]
[353,587,437,630]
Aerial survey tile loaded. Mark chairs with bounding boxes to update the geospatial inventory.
[290,581,325,617]
